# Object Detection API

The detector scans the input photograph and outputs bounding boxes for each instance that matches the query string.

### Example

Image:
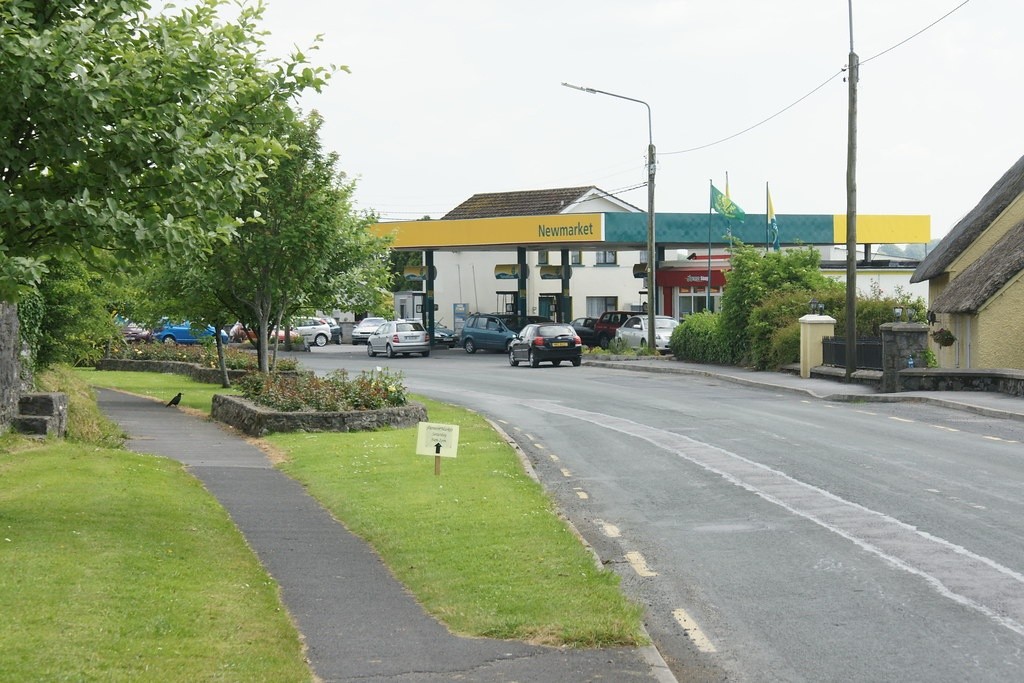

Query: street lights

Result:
[561,82,658,356]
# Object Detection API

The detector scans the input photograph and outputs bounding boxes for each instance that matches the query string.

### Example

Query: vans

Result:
[458,313,554,354]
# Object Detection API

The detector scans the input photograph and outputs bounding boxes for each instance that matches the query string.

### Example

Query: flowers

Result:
[929,327,956,342]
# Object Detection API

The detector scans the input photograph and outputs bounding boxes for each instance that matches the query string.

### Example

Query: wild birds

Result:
[166,392,184,408]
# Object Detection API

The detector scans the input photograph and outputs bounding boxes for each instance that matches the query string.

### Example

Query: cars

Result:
[153,316,229,348]
[507,321,583,368]
[116,317,150,342]
[569,317,613,348]
[351,316,388,346]
[614,314,683,355]
[366,320,431,358]
[404,317,460,348]
[228,312,344,351]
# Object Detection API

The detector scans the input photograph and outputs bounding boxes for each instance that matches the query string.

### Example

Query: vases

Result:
[932,338,954,346]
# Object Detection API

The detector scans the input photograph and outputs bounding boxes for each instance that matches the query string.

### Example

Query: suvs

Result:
[593,311,648,350]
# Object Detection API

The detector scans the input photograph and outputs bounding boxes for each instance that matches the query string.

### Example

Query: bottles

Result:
[908,354,914,368]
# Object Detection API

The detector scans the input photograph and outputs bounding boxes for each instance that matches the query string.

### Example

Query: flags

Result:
[720,184,732,238]
[712,185,745,221]
[767,189,780,252]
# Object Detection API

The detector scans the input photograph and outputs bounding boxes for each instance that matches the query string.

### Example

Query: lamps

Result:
[818,301,825,315]
[892,305,902,320]
[907,305,915,324]
[809,298,818,314]
